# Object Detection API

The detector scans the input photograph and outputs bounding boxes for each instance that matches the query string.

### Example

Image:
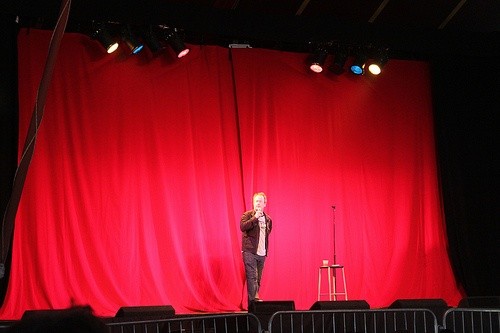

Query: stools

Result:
[318,266,348,302]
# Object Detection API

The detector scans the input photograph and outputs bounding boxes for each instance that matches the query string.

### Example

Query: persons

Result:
[240,192,272,302]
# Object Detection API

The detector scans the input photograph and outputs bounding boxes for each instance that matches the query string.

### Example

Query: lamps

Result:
[114,25,145,55]
[329,44,350,73]
[349,47,368,74]
[159,25,190,58]
[90,21,119,54]
[141,26,165,53]
[368,44,392,75]
[309,47,327,73]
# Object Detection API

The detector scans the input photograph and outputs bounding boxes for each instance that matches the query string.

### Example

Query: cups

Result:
[322,260,328,267]
